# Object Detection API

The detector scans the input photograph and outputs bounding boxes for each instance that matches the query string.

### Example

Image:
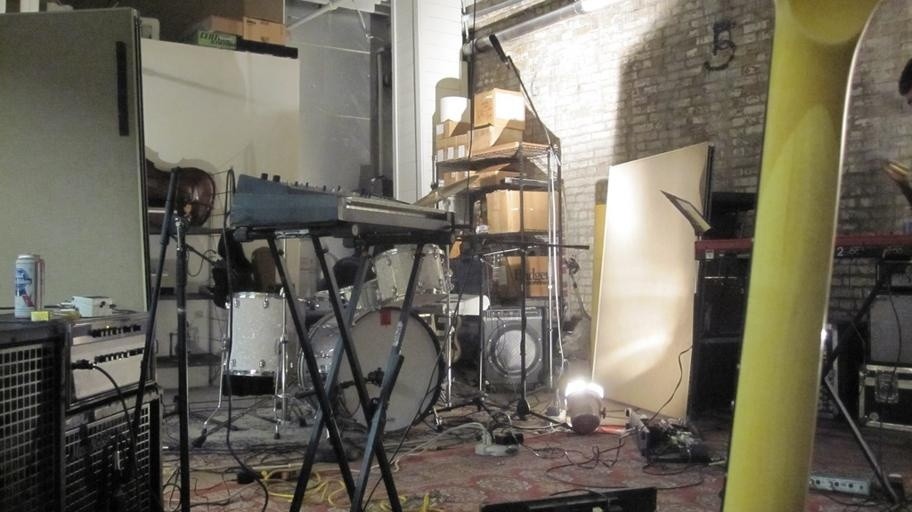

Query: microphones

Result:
[485,29,513,71]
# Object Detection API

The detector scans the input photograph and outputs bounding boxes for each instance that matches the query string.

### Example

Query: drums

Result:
[297,303,442,432]
[220,290,307,378]
[371,243,449,308]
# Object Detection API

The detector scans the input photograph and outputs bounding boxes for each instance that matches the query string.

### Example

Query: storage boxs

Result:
[241,16,288,45]
[497,256,551,297]
[436,139,445,161]
[472,126,523,150]
[486,190,551,234]
[857,363,911,431]
[474,88,526,129]
[446,136,457,160]
[183,16,244,36]
[186,28,236,50]
[469,163,520,188]
[435,119,471,139]
[456,134,468,160]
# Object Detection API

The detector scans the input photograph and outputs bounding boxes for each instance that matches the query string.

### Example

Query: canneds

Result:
[12,254,41,319]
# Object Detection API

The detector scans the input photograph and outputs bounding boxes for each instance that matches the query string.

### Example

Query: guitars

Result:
[338,279,376,308]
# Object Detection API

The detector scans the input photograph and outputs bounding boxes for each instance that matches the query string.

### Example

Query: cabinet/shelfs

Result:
[433,141,555,397]
[147,169,237,389]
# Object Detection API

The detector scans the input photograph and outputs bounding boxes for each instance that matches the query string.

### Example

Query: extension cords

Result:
[470,437,520,459]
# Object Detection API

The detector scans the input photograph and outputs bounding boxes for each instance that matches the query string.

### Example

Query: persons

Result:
[881,59,912,211]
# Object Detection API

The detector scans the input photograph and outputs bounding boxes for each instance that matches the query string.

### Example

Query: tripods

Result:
[431,260,511,433]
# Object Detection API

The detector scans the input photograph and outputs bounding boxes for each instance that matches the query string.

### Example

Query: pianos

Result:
[230,173,454,237]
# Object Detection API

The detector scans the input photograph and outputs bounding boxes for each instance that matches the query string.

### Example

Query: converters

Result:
[810,465,877,497]
